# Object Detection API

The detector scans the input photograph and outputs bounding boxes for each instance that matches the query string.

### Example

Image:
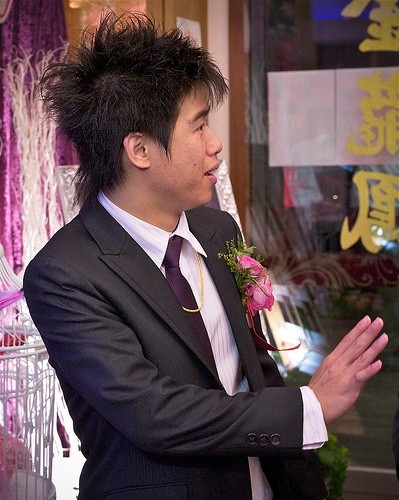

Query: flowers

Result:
[217,233,276,317]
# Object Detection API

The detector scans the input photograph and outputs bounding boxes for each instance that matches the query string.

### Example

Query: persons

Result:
[23,10,388,500]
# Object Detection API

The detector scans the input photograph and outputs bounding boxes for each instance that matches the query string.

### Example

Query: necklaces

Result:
[182,250,204,313]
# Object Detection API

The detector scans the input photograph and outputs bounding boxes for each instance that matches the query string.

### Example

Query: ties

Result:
[163,235,215,365]
